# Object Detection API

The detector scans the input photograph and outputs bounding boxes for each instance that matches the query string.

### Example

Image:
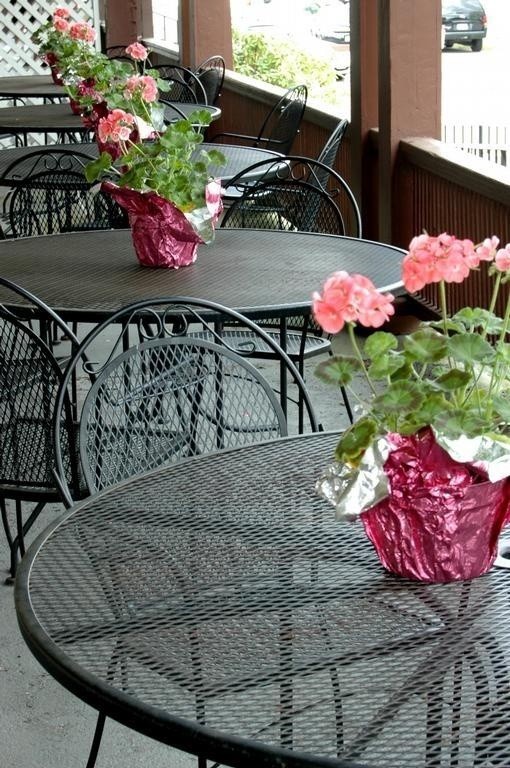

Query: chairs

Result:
[52,294,447,768]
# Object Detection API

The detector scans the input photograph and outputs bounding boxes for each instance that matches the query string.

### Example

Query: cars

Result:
[240,1,487,78]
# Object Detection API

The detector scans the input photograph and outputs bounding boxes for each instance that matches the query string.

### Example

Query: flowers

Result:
[33,8,97,57]
[311,223,510,469]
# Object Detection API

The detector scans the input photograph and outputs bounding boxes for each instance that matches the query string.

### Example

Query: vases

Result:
[322,431,510,584]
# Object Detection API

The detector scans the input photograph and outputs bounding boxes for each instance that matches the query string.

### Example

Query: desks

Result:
[0,226,419,576]
[13,427,510,767]
